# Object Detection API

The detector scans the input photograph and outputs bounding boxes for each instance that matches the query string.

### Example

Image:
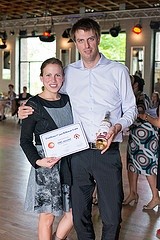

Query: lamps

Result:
[11,30,14,36]
[133,18,142,34]
[45,26,52,36]
[150,18,160,29]
[62,28,71,38]
[110,20,121,37]
[19,27,27,35]
[31,27,39,36]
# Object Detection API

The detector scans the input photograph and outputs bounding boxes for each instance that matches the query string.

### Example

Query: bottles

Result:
[96,111,112,150]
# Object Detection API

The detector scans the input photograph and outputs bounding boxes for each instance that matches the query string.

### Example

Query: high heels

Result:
[143,199,160,210]
[122,193,139,205]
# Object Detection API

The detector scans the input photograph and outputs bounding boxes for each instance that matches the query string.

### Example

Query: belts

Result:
[87,142,119,150]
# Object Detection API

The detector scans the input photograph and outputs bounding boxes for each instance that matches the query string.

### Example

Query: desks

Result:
[0,100,11,120]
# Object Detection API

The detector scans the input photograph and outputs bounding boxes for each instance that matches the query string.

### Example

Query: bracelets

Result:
[145,114,150,122]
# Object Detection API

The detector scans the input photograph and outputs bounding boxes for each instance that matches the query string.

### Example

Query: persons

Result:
[156,229,160,239]
[17,86,32,107]
[19,58,74,240]
[123,70,160,210]
[6,83,16,105]
[17,16,138,240]
